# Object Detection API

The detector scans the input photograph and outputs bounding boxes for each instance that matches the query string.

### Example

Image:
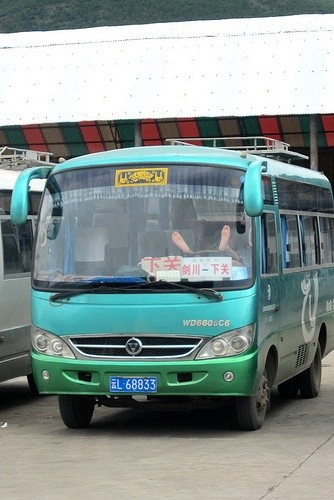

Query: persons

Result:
[171,224,248,262]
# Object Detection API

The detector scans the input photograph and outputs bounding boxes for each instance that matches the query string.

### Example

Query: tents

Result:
[0,12,334,173]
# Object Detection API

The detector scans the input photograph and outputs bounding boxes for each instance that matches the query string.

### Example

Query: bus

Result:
[8,134,334,433]
[0,146,65,400]
